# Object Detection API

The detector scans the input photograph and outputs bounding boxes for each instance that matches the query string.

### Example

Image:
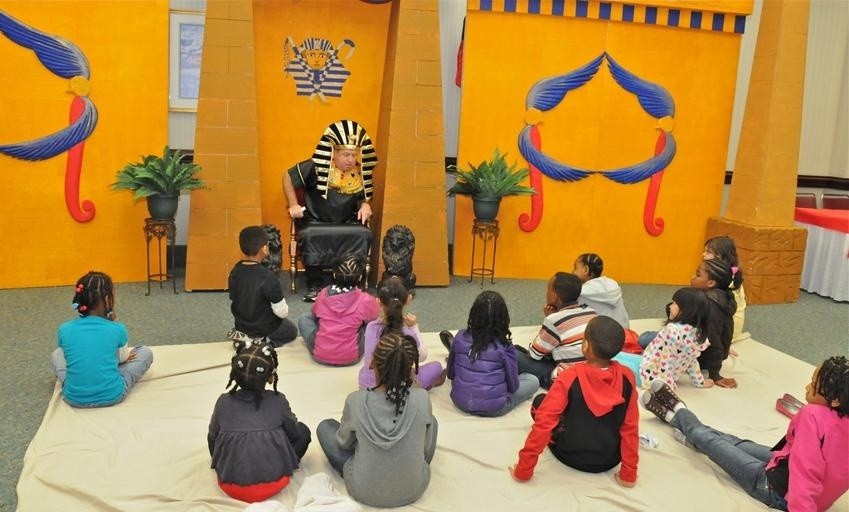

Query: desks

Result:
[793,206,848,303]
[143,217,179,297]
[467,218,500,290]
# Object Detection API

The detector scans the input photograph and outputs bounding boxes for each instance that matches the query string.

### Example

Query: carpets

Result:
[14,314,849,512]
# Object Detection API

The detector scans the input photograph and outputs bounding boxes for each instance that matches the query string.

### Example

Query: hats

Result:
[312,120,377,203]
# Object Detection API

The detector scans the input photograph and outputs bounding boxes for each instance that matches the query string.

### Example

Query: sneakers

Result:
[302,286,322,302]
[227,328,271,350]
[639,379,686,424]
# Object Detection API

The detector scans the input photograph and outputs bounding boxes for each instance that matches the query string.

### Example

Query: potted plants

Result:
[107,144,208,222]
[445,143,538,222]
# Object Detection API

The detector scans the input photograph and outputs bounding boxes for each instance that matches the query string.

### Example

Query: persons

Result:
[511,271,596,390]
[227,226,298,352]
[283,119,378,303]
[609,287,714,395]
[297,256,447,508]
[638,260,737,388]
[52,272,153,410]
[207,338,313,503]
[638,356,849,511]
[509,316,639,488]
[447,291,539,420]
[573,253,630,343]
[702,236,746,357]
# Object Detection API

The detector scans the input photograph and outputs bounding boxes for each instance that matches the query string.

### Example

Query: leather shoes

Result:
[777,393,805,419]
[440,330,455,352]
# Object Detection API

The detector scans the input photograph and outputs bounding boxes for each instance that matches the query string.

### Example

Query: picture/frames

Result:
[167,7,206,114]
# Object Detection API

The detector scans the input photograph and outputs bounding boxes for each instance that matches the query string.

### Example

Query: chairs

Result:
[819,192,848,210]
[289,185,373,296]
[795,191,817,209]
